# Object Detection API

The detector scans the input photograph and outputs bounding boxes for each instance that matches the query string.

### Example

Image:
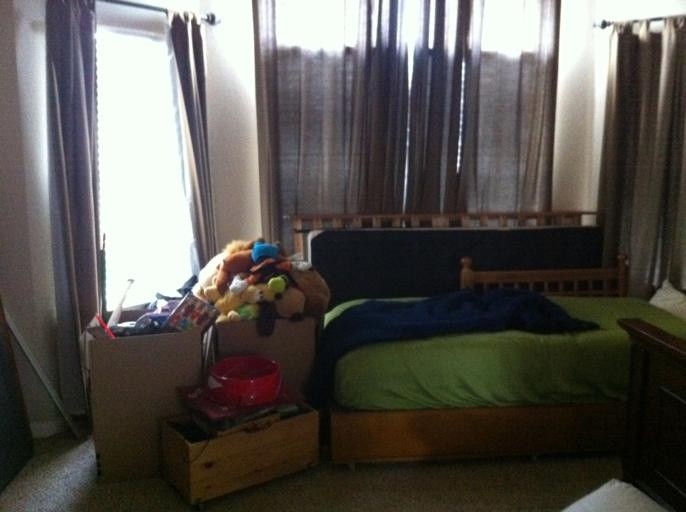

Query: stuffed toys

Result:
[192,239,332,324]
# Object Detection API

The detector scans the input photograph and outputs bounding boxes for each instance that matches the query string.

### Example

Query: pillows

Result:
[648,277,686,321]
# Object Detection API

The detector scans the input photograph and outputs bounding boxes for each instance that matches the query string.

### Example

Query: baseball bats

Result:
[107,278,134,329]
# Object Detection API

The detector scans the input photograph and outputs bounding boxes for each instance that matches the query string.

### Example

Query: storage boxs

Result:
[82,320,204,484]
[161,398,320,506]
[203,315,317,399]
[102,307,156,325]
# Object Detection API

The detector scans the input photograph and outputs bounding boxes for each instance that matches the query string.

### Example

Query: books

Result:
[163,292,221,337]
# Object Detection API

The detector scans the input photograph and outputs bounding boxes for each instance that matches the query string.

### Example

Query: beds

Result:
[323,293,686,464]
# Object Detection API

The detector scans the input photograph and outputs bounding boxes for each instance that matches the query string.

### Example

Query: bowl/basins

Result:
[209,355,283,403]
